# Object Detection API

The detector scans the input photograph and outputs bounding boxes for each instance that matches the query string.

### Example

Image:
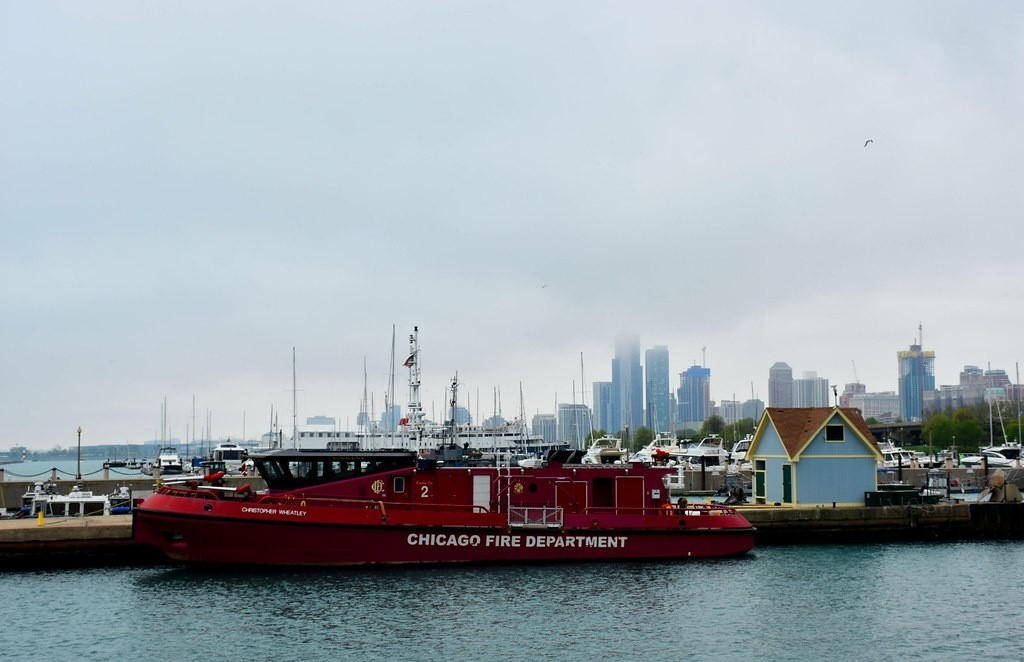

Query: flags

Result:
[404,354,415,368]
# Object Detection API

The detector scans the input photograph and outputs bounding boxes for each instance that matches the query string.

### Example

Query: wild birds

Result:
[864,138,875,147]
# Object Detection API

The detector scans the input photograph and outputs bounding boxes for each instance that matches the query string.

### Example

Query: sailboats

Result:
[1,322,1023,567]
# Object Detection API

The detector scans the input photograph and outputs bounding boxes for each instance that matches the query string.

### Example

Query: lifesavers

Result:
[658,504,676,515]
[280,494,298,505]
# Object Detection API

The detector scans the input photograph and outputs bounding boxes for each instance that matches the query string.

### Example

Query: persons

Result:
[739,487,743,500]
[932,479,937,487]
[208,468,228,498]
[20,500,34,516]
[716,485,727,496]
[967,480,971,486]
